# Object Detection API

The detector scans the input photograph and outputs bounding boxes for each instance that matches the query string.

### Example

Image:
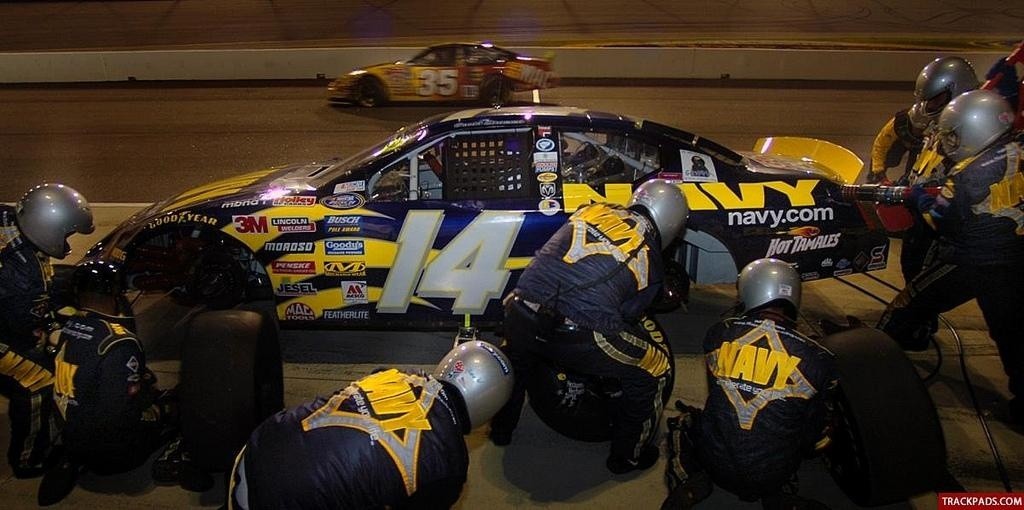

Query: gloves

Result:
[908,184,936,211]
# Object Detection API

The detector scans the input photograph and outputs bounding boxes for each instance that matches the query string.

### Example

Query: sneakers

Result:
[764,489,829,509]
[660,474,715,509]
[607,443,660,474]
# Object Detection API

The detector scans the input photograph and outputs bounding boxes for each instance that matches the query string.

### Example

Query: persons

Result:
[427,48,452,65]
[861,49,986,355]
[31,261,206,510]
[659,256,858,508]
[870,53,1023,437]
[0,182,95,482]
[484,176,689,477]
[223,341,512,509]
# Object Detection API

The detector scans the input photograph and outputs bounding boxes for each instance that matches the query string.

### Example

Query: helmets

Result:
[68,260,127,296]
[736,256,802,322]
[913,55,981,123]
[625,179,690,252]
[434,341,515,436]
[937,89,1014,163]
[17,184,94,261]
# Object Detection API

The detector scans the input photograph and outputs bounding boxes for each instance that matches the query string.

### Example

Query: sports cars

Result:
[73,103,893,335]
[325,40,556,109]
